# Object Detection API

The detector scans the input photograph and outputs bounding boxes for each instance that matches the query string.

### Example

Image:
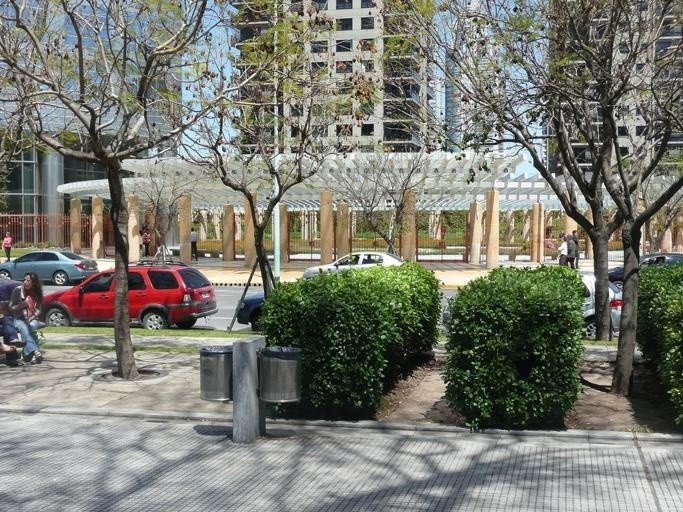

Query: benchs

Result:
[0,301,47,339]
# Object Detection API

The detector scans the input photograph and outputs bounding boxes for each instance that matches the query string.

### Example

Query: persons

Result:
[1,231,13,262]
[142,229,152,257]
[190,227,197,261]
[545,228,621,270]
[138,230,143,250]
[0,271,46,367]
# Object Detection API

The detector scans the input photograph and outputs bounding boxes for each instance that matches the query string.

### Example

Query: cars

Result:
[0,277,23,315]
[0,250,99,286]
[234,294,265,333]
[606,253,682,291]
[300,251,405,279]
[576,271,623,342]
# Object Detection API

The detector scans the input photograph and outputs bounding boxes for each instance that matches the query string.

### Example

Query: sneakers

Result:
[30,352,43,364]
[0,345,16,354]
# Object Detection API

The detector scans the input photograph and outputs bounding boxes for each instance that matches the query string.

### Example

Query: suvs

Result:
[36,260,218,331]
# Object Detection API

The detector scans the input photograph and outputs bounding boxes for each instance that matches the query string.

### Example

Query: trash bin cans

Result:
[200,336,302,443]
